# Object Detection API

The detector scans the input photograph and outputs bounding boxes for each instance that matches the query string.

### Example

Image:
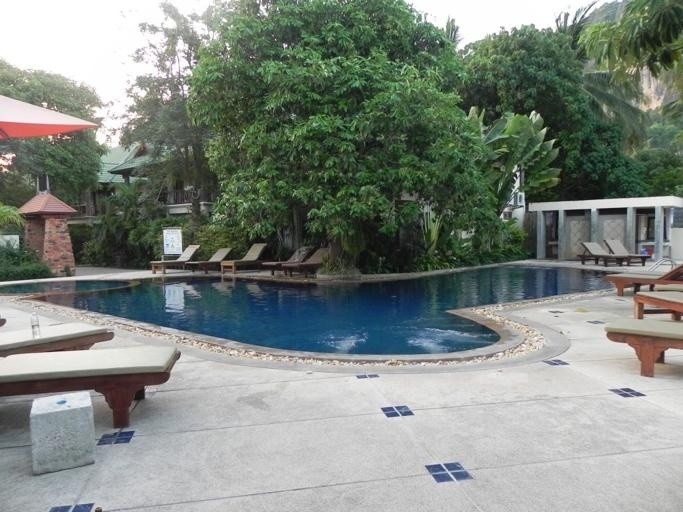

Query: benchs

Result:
[602,282,682,377]
[0,320,182,428]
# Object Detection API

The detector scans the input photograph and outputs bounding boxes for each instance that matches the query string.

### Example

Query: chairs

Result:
[575,239,651,267]
[601,263,682,297]
[150,242,330,276]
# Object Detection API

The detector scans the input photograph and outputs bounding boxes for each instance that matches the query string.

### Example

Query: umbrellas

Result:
[0,94,99,140]
